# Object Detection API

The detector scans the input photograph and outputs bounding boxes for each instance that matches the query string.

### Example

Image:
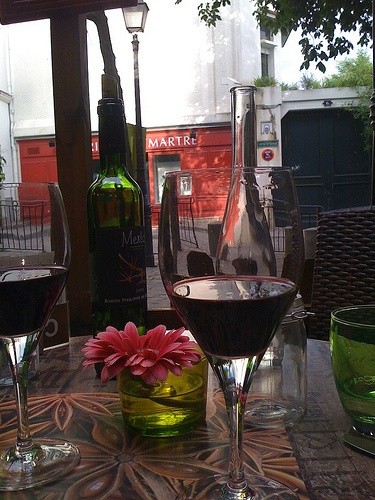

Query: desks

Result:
[0,336,375,500]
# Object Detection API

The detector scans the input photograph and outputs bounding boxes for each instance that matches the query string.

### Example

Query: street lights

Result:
[122,1,156,266]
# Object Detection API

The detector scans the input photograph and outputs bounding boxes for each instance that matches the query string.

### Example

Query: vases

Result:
[119,343,208,438]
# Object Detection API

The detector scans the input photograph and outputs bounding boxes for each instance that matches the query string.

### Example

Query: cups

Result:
[328,302,374,440]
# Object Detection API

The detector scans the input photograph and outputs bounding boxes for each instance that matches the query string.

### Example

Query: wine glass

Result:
[0,181,82,494]
[158,169,303,500]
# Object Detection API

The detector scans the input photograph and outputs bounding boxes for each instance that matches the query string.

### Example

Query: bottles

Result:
[210,82,288,430]
[88,98,149,379]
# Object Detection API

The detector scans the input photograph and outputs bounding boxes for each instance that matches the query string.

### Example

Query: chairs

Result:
[306,207,375,343]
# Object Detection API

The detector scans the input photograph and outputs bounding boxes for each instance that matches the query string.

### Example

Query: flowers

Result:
[81,323,199,384]
